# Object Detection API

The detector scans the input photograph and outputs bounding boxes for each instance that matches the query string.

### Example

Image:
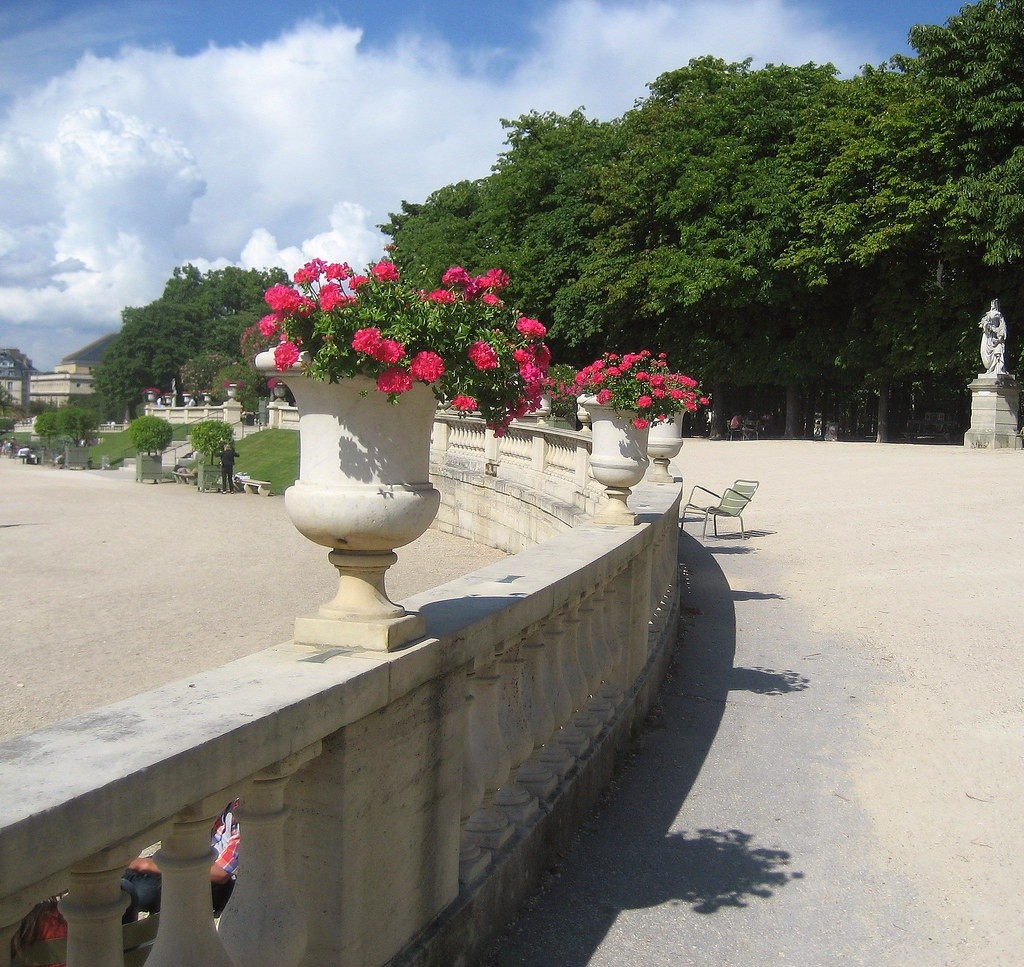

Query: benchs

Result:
[232,475,271,498]
[727,419,760,440]
[172,470,197,484]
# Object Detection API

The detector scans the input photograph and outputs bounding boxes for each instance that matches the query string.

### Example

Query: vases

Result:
[273,383,287,402]
[648,398,689,485]
[587,396,650,525]
[147,391,192,407]
[202,393,212,406]
[227,384,239,402]
[254,345,441,653]
[577,394,593,433]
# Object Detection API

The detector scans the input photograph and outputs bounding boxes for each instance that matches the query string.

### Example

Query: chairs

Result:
[679,479,759,540]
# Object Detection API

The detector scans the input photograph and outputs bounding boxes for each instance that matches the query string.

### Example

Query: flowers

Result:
[259,258,551,439]
[202,389,212,393]
[144,387,161,395]
[223,378,245,390]
[266,377,282,390]
[534,392,553,429]
[544,364,710,429]
[164,392,173,396]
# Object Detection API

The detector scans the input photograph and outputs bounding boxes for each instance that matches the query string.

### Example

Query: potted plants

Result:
[191,420,234,492]
[129,415,173,483]
[35,408,99,470]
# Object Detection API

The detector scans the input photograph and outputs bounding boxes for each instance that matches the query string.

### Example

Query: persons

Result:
[174,464,198,474]
[980,299,1008,374]
[14,798,243,944]
[218,443,240,494]
[0,436,32,463]
[731,412,752,440]
